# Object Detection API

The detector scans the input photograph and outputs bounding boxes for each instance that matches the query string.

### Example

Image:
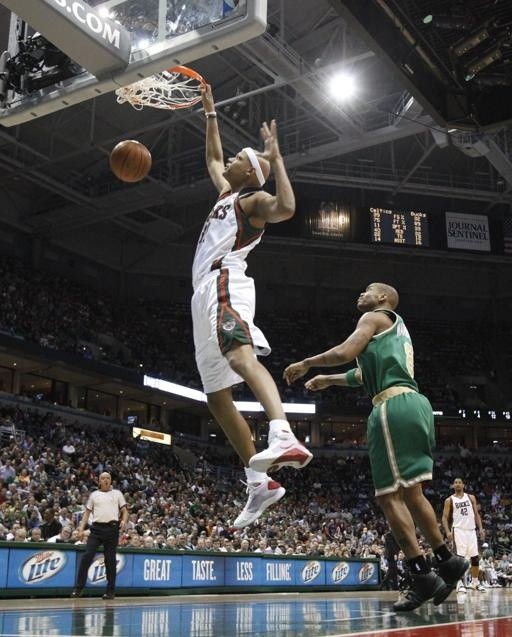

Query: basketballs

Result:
[110,139,151,182]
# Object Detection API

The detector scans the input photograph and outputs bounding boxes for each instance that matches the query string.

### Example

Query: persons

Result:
[441,475,486,594]
[1,219,511,585]
[282,280,469,616]
[189,73,315,532]
[68,466,130,599]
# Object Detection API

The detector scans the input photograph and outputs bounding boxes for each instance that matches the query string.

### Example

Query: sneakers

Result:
[393,554,469,611]
[233,478,286,528]
[248,433,313,472]
[72,591,82,597]
[102,592,115,599]
[475,583,487,593]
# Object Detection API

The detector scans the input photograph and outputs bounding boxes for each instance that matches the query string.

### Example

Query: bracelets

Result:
[203,111,218,118]
